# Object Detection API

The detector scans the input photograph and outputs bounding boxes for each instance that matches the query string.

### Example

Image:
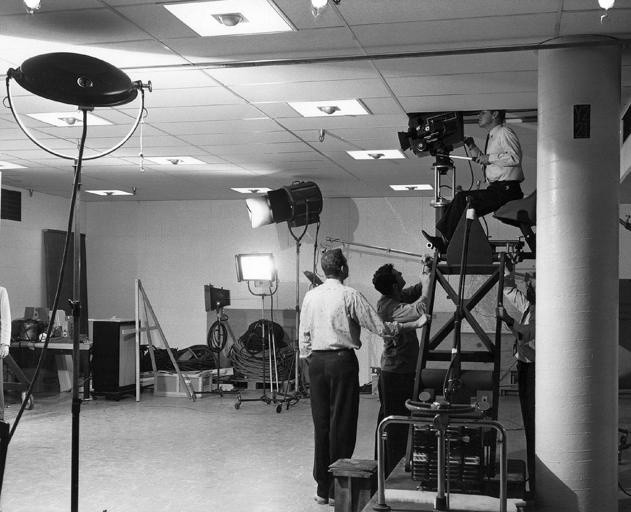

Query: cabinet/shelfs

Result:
[87,318,143,405]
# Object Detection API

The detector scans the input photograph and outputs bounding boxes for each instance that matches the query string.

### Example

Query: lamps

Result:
[233,251,288,412]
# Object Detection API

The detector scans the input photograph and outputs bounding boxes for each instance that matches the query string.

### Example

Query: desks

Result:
[4,334,94,399]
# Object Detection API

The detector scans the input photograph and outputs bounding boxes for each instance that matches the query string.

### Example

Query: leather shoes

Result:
[422,230,446,255]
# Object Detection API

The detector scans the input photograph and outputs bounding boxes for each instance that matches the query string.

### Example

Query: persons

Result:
[297,247,431,505]
[0,285,14,422]
[372,261,431,489]
[420,109,524,254]
[497,257,536,498]
